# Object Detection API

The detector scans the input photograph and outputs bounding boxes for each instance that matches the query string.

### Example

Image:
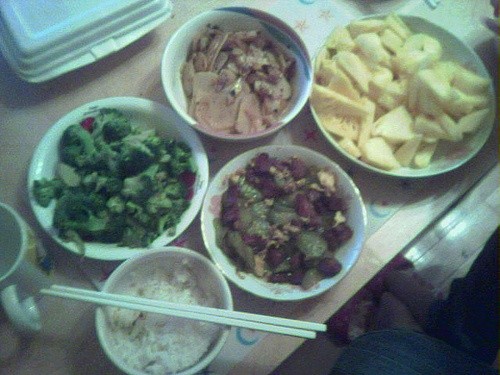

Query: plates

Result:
[28,96,208,260]
[200,143,367,301]
[309,13,497,178]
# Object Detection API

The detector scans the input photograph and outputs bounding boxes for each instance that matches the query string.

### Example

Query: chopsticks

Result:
[40,284,327,339]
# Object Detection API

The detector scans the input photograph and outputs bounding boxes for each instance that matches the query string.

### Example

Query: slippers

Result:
[367,254,443,309]
[324,288,383,344]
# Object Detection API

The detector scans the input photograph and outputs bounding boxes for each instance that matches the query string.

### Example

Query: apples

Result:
[312,10,491,171]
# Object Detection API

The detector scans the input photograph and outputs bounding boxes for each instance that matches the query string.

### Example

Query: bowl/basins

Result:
[163,7,313,141]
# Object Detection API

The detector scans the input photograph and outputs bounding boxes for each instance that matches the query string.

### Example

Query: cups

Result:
[0,204,54,337]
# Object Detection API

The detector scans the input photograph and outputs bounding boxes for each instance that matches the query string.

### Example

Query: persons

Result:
[329,224,499,374]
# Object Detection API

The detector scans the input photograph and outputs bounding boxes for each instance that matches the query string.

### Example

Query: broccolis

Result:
[26,97,208,261]
